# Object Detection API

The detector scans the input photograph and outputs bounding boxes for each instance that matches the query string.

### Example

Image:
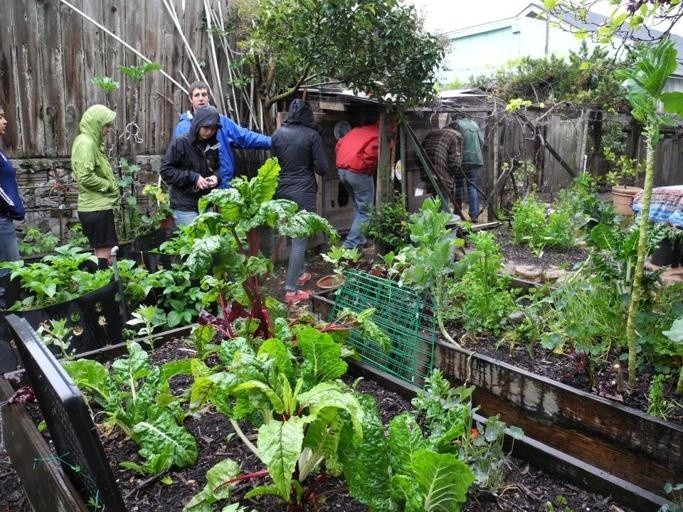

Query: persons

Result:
[452,112,484,224]
[415,122,462,213]
[0,107,26,314]
[70,103,119,265]
[271,97,328,304]
[156,105,222,233]
[334,111,396,251]
[174,80,271,189]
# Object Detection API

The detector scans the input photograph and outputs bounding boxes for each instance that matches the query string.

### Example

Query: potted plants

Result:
[0,229,119,350]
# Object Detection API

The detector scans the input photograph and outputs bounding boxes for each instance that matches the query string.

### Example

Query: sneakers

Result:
[297,272,311,286]
[285,289,314,303]
